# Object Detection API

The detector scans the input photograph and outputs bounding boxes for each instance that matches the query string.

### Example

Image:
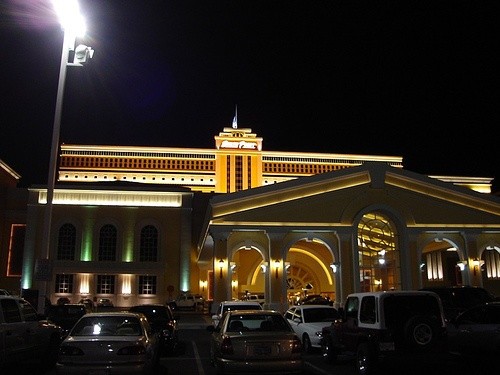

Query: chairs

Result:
[261,321,272,327]
[231,321,243,329]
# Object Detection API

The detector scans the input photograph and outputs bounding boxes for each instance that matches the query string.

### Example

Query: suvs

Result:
[1,289,63,367]
[416,285,496,324]
[319,291,446,375]
[167,293,204,311]
[127,305,178,350]
[45,303,87,329]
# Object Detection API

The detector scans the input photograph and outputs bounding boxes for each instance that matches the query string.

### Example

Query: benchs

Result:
[79,326,134,334]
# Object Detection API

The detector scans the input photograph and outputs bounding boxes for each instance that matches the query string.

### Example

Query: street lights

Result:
[37,0,95,313]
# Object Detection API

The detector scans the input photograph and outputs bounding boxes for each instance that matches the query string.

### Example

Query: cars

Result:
[283,304,342,353]
[210,309,302,375]
[211,301,272,331]
[447,296,500,364]
[96,298,113,309]
[63,312,156,375]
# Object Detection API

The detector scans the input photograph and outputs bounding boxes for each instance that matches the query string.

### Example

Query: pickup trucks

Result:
[238,294,264,305]
